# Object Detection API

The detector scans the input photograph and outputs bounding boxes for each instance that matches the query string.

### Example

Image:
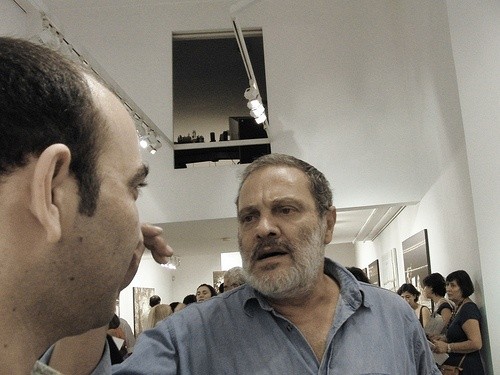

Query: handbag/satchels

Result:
[441,364,463,375]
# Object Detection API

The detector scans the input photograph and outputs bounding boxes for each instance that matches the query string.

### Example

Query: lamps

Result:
[243,79,259,101]
[249,104,266,119]
[255,113,267,125]
[246,99,262,110]
[160,255,182,270]
[24,13,163,156]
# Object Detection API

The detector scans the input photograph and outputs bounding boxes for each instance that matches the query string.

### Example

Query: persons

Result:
[0,36,149,375]
[40,154,443,375]
[396,283,431,328]
[430,270,485,375]
[423,273,455,334]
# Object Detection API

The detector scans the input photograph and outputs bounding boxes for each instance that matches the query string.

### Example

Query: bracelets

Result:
[452,343,456,353]
[447,343,451,353]
